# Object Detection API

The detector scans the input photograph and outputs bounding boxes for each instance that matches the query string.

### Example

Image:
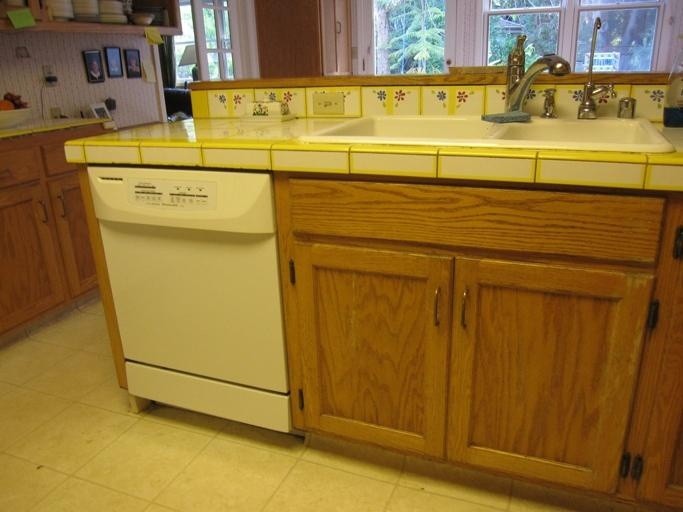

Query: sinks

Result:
[297,114,496,153]
[490,113,675,154]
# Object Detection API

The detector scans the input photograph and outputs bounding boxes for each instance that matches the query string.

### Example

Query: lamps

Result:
[178,45,199,81]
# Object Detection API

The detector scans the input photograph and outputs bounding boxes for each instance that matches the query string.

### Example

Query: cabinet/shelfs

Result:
[0,0,182,35]
[254,0,353,79]
[627,192,683,512]
[0,124,116,348]
[274,172,674,512]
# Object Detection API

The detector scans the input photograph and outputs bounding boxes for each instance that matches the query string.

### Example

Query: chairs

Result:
[165,88,192,120]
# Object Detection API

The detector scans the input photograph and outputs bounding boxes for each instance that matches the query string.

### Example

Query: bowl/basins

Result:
[0,108,33,130]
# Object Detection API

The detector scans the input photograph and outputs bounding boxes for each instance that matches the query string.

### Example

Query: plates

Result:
[2,0,165,25]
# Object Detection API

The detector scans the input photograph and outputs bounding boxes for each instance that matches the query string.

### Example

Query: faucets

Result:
[506,32,573,113]
[577,15,617,120]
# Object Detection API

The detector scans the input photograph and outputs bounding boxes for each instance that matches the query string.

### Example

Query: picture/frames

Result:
[82,47,142,83]
[90,102,115,129]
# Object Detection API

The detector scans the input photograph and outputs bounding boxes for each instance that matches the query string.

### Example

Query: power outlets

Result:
[43,65,57,86]
